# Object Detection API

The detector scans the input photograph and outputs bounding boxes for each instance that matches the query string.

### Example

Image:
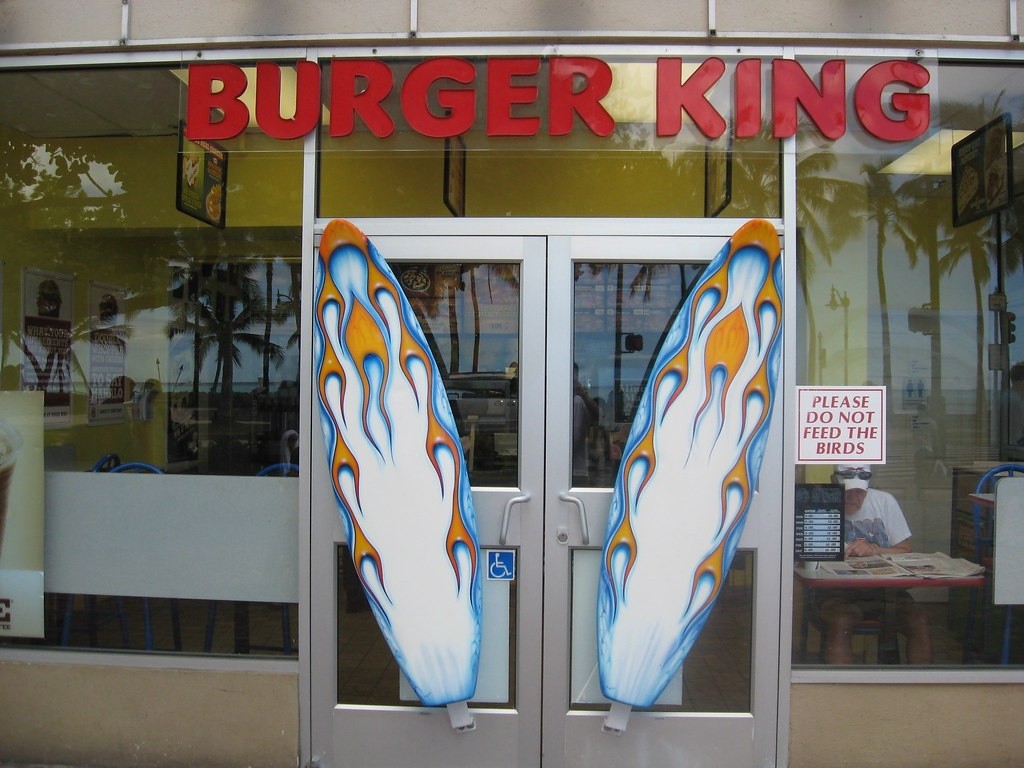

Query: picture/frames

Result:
[172,262,252,324]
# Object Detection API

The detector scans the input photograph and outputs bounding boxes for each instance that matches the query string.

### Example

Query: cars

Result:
[442,370,589,466]
[607,380,646,446]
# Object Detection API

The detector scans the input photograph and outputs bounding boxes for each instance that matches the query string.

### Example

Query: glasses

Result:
[834,470,872,480]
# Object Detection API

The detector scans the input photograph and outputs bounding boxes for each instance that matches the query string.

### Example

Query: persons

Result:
[572,362,591,487]
[810,462,932,665]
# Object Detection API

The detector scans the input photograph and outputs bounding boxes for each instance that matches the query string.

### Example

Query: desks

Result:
[461,416,517,474]
[794,556,986,664]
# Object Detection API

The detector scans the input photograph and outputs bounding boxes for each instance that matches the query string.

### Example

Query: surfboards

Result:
[310,219,488,735]
[593,218,786,739]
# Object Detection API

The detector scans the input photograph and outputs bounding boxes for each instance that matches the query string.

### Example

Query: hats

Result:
[833,463,871,492]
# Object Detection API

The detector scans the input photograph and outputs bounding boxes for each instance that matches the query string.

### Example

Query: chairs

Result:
[962,463,1024,664]
[799,583,888,665]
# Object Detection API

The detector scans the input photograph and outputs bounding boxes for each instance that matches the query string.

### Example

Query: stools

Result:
[58,453,300,654]
[947,465,1023,664]
